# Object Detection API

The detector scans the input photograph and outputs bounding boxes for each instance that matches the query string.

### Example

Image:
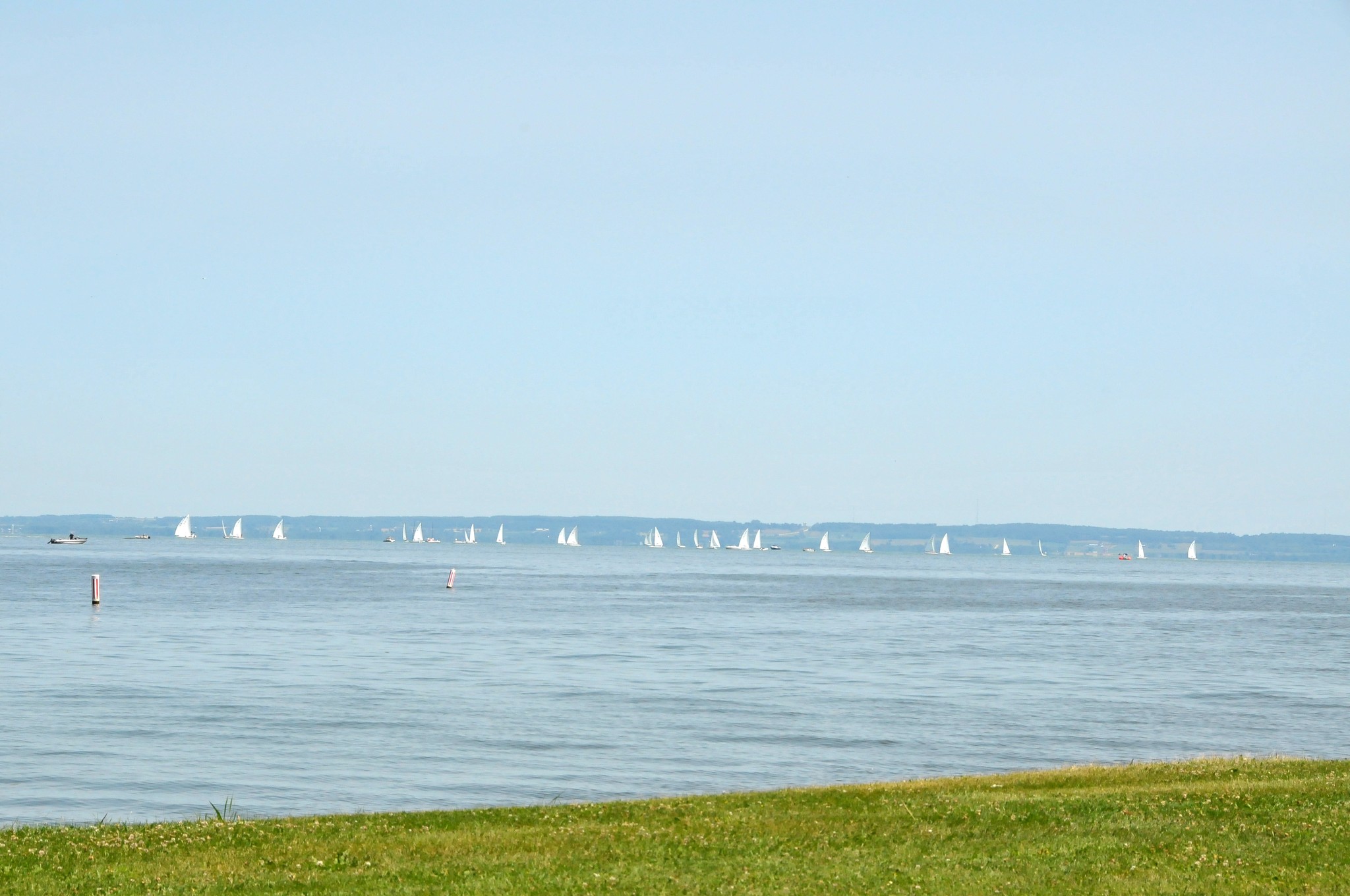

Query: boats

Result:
[135,533,148,539]
[47,536,88,544]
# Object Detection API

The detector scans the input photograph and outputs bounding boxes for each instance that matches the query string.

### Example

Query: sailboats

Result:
[175,515,1202,562]
